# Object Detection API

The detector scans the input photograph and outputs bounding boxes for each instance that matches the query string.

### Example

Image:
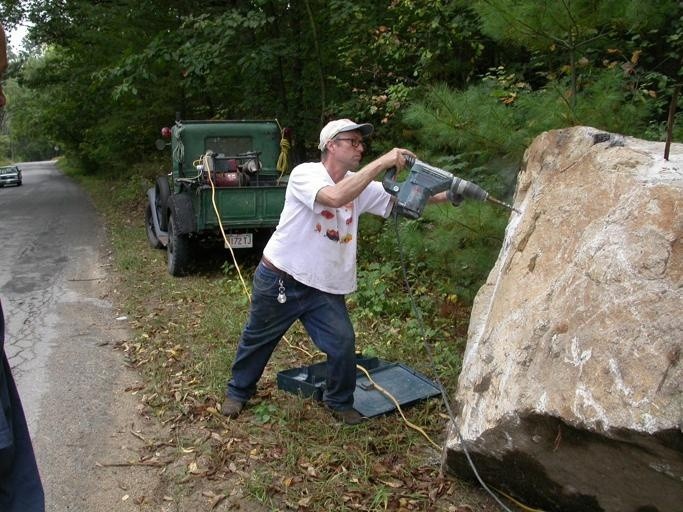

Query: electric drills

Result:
[383,153,523,220]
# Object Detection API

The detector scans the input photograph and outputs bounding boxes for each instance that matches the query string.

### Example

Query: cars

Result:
[0,165,23,188]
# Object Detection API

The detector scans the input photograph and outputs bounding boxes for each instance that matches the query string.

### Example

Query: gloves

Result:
[446,179,474,207]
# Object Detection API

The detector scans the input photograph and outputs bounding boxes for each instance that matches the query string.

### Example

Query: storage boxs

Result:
[277,355,442,420]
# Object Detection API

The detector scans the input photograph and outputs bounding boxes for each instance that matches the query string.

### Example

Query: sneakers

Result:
[221,395,247,421]
[323,401,363,425]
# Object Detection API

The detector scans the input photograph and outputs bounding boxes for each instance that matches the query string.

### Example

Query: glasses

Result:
[334,138,365,148]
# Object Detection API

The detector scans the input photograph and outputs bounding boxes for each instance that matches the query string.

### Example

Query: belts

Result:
[261,252,286,273]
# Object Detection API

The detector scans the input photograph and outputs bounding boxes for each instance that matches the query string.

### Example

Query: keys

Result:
[277,280,286,304]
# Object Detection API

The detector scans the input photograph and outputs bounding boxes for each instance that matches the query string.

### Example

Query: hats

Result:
[317,118,375,152]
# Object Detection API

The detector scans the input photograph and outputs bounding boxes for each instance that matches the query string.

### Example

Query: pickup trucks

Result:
[142,117,295,284]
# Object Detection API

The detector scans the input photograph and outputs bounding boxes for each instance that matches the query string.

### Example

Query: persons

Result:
[0,28,46,512]
[221,119,448,424]
